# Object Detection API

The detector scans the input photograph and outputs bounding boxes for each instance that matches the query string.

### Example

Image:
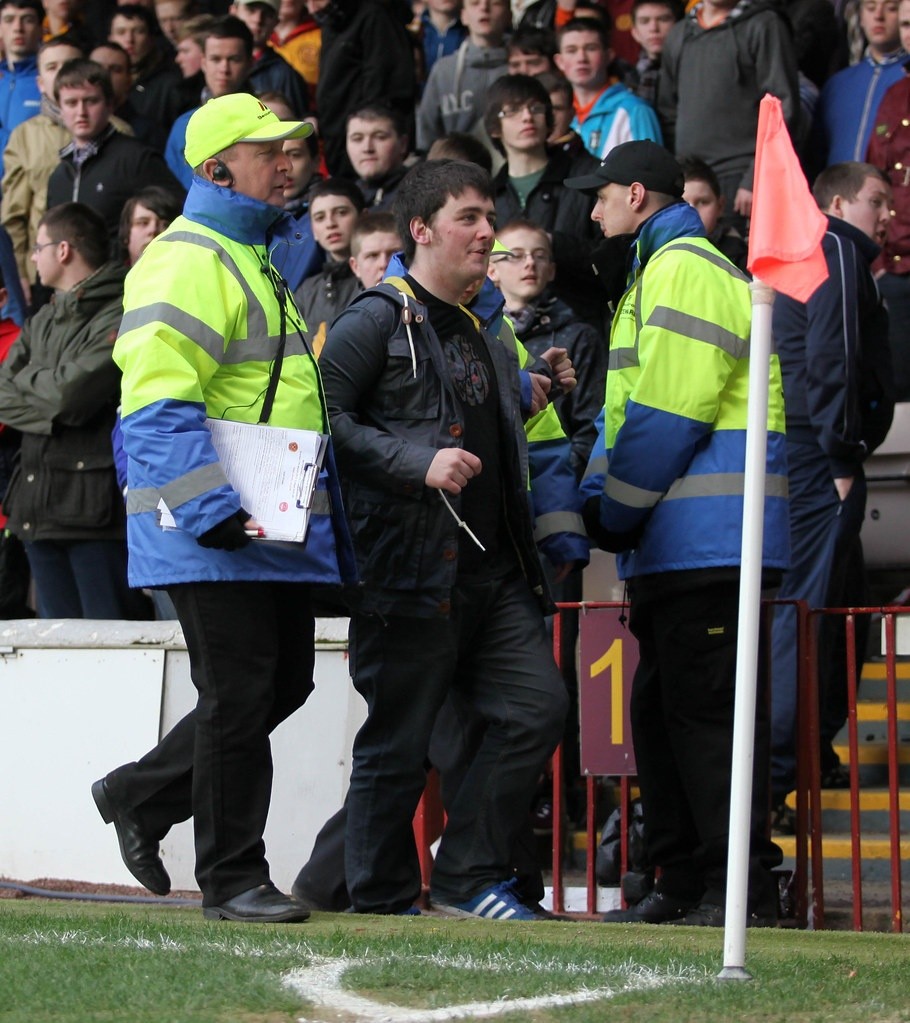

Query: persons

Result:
[578,137,795,926]
[0,0,910,911]
[93,88,358,919]
[289,155,578,921]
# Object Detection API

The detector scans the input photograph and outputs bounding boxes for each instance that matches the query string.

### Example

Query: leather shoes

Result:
[91,777,171,896]
[204,884,311,922]
[603,889,778,928]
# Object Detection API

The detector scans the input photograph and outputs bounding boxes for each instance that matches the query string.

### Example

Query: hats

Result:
[563,138,686,197]
[184,93,314,169]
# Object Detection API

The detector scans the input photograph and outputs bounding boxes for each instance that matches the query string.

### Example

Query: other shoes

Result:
[820,765,872,789]
[526,903,576,922]
[291,885,327,911]
[772,804,795,834]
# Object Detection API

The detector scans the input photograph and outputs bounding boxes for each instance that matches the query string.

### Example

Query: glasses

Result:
[33,241,79,254]
[492,250,551,264]
[496,104,547,118]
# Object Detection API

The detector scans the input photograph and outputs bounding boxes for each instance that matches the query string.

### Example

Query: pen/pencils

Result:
[244,529,264,537]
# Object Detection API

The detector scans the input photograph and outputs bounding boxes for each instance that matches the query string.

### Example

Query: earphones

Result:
[213,161,227,181]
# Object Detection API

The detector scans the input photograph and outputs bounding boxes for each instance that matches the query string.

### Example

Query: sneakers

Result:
[430,877,545,921]
